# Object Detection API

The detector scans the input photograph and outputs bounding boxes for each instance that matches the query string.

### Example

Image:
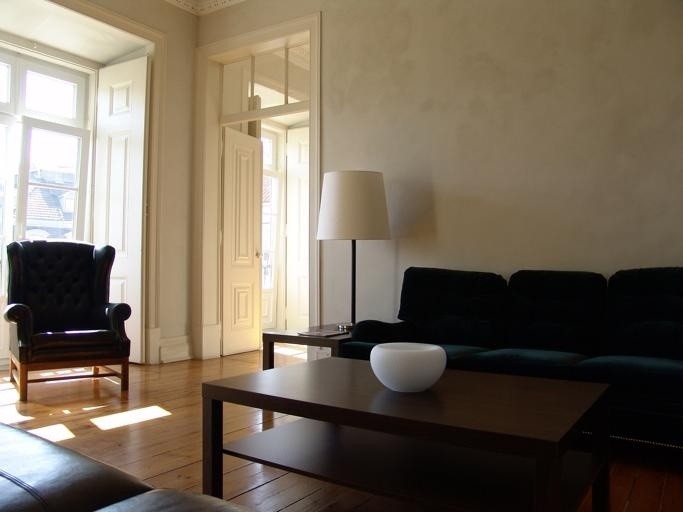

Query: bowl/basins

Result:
[369,342,448,394]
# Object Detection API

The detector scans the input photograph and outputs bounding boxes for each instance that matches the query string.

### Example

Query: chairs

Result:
[3,239,132,403]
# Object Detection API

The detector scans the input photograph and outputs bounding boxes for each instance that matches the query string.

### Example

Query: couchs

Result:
[340,263,682,470]
[0,422,241,511]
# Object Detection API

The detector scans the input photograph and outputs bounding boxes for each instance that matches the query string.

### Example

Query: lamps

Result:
[311,168,394,328]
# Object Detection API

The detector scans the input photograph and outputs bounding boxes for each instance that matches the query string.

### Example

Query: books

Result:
[299,326,349,339]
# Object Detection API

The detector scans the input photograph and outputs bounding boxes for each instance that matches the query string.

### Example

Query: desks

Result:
[257,324,354,370]
